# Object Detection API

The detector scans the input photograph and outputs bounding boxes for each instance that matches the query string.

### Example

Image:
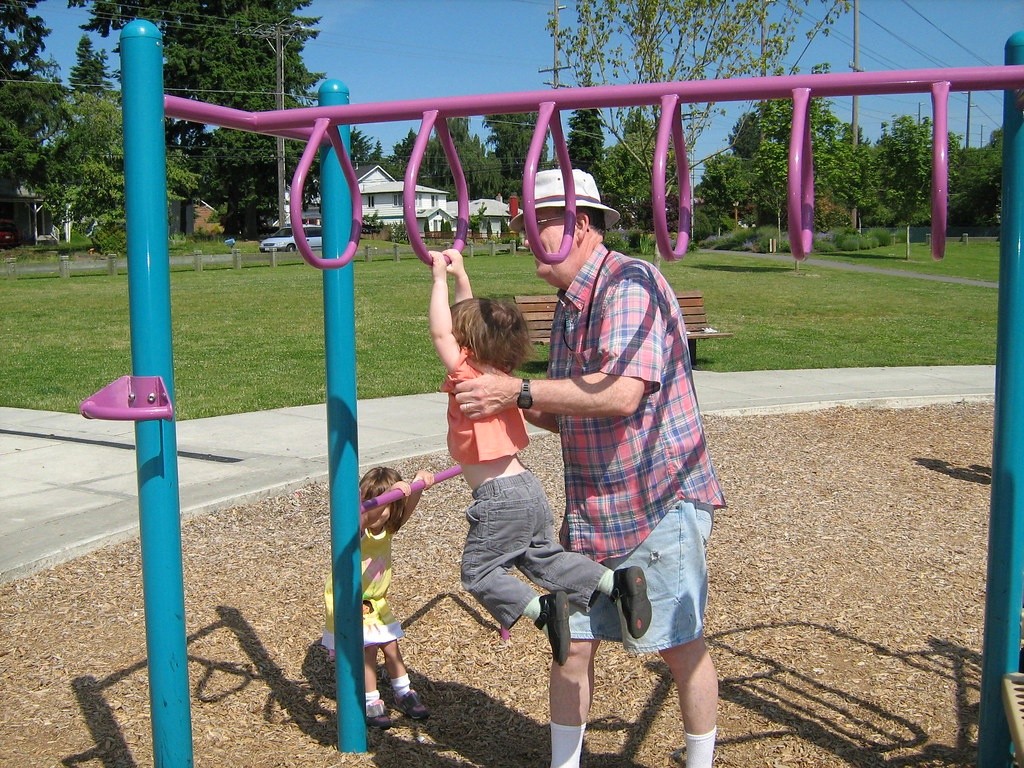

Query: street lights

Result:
[732,200,740,233]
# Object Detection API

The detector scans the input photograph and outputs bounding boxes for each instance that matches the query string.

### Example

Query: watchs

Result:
[516,379,533,409]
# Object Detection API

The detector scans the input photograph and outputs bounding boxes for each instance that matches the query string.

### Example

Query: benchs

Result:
[514,290,734,370]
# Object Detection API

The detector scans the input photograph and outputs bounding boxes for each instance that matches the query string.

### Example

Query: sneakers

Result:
[393,689,430,719]
[366,699,392,727]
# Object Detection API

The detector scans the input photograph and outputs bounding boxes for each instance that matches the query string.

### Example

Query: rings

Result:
[464,404,467,412]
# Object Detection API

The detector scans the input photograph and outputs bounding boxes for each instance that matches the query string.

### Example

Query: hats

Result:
[509,169,620,233]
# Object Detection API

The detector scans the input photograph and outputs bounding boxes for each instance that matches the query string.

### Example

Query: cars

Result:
[0,217,22,250]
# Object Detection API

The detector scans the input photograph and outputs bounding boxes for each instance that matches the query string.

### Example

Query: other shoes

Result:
[609,566,652,639]
[534,590,571,666]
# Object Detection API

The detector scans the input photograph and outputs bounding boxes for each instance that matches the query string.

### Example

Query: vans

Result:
[258,222,322,254]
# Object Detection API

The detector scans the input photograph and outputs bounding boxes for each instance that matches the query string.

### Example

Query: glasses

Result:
[524,216,564,232]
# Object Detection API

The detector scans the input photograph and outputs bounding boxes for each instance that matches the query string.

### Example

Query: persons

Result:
[321,467,434,729]
[456,167,730,768]
[429,248,653,666]
